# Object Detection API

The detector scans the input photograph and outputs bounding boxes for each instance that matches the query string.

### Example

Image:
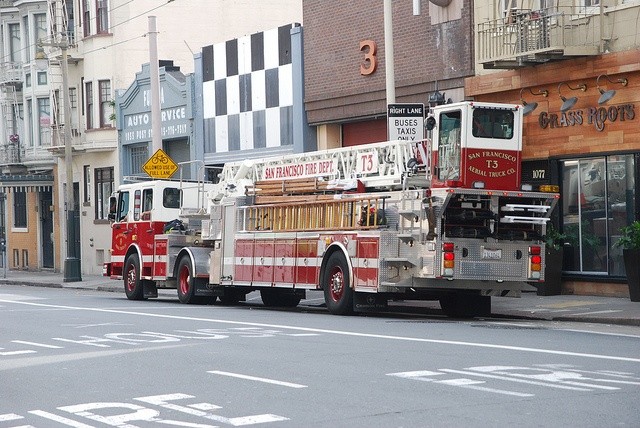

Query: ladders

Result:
[236,195,391,233]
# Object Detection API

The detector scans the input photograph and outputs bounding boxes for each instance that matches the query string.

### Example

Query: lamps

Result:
[519,86,549,115]
[595,74,628,105]
[557,80,587,111]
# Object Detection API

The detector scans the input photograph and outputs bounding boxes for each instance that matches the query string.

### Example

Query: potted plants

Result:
[611,221,640,302]
[565,218,601,270]
[537,226,566,295]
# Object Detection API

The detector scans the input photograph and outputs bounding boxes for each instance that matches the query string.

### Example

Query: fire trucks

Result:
[102,100,562,316]
